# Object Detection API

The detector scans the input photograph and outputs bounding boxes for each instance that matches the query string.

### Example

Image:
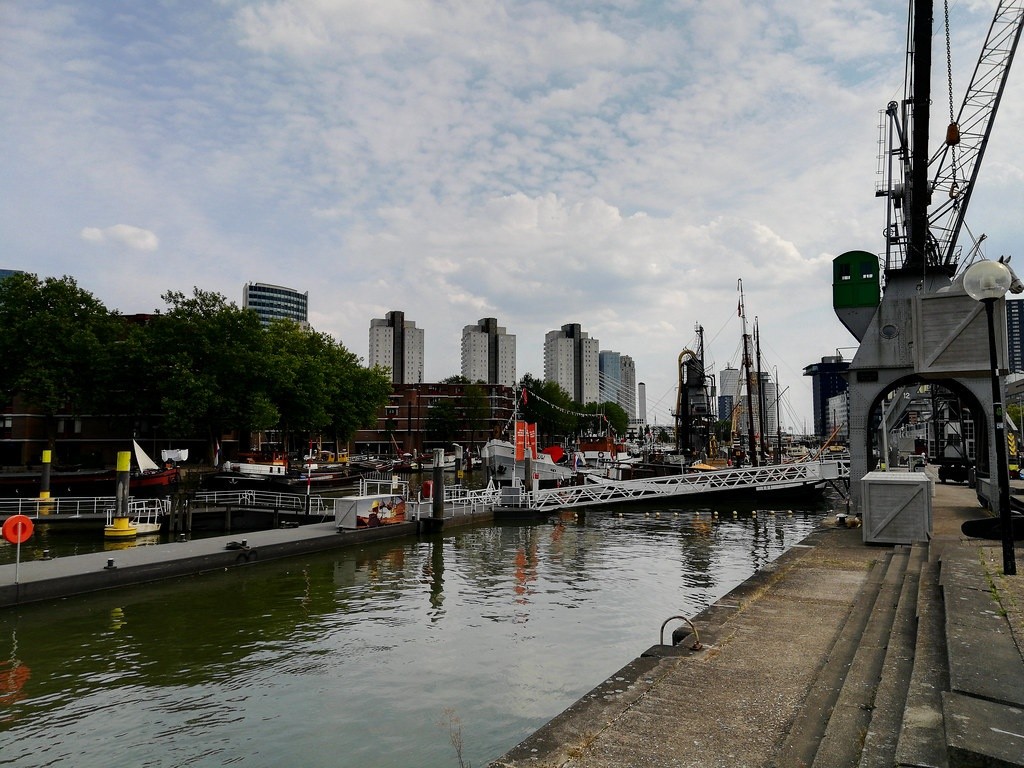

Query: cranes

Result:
[825,0,1024,524]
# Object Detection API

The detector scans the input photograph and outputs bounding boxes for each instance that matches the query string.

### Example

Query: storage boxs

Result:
[860,471,933,543]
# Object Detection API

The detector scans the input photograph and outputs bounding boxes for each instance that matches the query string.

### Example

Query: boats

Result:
[479,437,573,486]
[302,435,484,472]
[105,505,162,536]
[570,274,847,489]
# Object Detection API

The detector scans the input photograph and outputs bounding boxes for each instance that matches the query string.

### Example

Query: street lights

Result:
[960,260,1022,576]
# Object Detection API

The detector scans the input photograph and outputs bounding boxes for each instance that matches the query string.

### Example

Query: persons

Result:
[368,501,380,527]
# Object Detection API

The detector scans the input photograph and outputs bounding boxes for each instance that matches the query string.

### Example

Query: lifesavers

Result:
[598,452,604,458]
[582,452,585,457]
